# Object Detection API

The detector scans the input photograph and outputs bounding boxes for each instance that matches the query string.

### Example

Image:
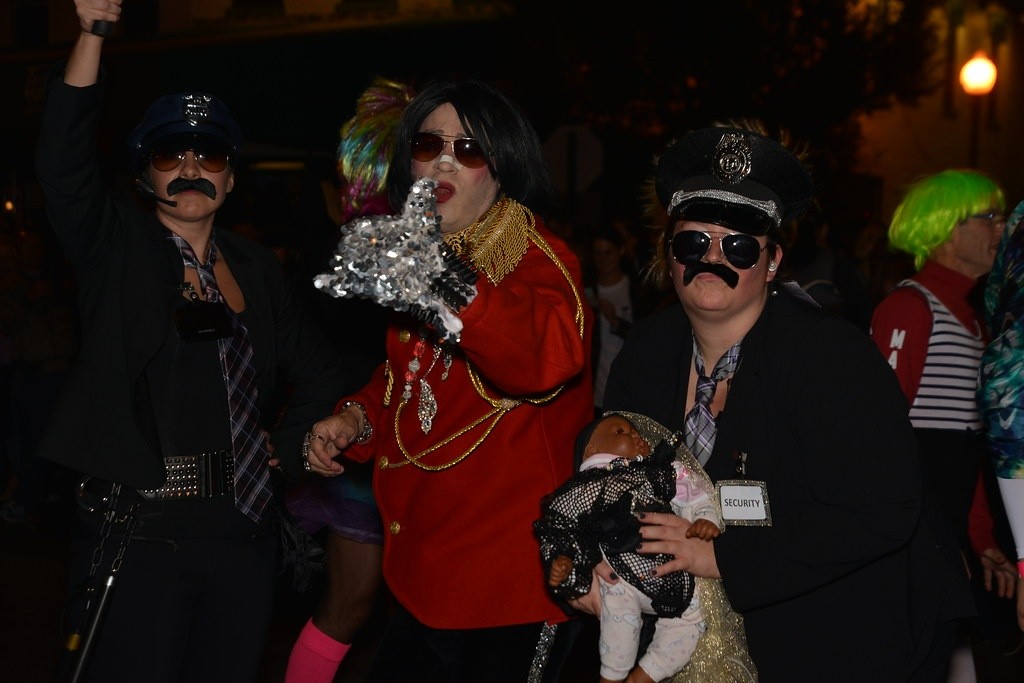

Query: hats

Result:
[126,91,243,165]
[655,129,819,252]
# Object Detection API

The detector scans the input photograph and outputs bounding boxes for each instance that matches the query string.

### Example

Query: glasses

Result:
[972,210,1002,226]
[664,229,768,269]
[144,135,230,173]
[409,131,491,169]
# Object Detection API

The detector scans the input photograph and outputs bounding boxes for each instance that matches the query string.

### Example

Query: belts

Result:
[134,446,234,504]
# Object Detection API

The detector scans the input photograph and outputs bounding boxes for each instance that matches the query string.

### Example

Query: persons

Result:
[0,228,71,525]
[600,127,925,683]
[535,413,724,683]
[584,225,647,417]
[870,170,1019,683]
[34,1,349,683]
[283,79,416,682]
[301,81,597,682]
[980,201,1024,636]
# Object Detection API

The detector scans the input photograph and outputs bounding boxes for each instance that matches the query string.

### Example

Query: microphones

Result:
[135,175,177,207]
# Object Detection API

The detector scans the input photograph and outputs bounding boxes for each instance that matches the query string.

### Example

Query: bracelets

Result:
[1017,561,1024,578]
[342,402,373,443]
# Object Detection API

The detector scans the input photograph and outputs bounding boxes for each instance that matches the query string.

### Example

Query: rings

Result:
[304,461,312,472]
[305,433,325,441]
[302,441,311,456]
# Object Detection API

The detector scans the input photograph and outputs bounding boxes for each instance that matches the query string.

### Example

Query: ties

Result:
[177,228,279,525]
[687,334,741,467]
